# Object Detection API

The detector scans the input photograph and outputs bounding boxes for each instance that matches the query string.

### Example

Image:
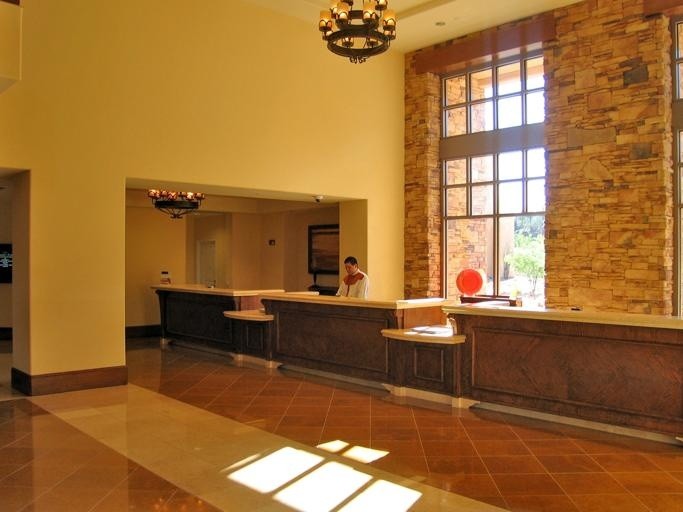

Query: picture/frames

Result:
[307,224,338,276]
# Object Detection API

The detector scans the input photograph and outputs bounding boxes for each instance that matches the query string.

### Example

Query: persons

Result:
[336,256,368,299]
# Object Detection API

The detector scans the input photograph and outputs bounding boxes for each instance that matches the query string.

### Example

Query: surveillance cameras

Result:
[315,196,324,203]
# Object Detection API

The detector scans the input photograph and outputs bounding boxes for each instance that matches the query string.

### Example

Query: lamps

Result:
[147,188,204,223]
[318,0,396,65]
